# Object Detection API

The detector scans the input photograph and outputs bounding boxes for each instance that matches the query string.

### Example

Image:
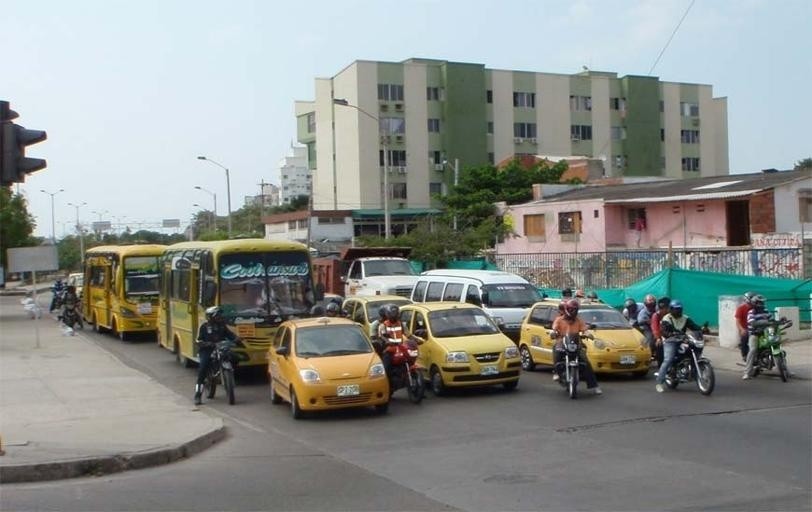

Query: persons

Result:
[370,305,386,354]
[575,290,584,298]
[623,299,645,326]
[327,303,341,317]
[656,300,711,393]
[562,288,572,298]
[194,306,241,405]
[552,301,587,374]
[550,300,603,395]
[587,291,597,299]
[50,276,85,329]
[651,297,671,370]
[379,303,424,375]
[742,294,796,380]
[735,291,756,363]
[330,297,350,318]
[633,217,645,248]
[310,304,326,318]
[637,295,660,360]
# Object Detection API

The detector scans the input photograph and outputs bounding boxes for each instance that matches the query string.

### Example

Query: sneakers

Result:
[656,384,663,393]
[194,395,201,405]
[789,371,795,376]
[552,374,559,381]
[742,373,748,379]
[594,387,602,394]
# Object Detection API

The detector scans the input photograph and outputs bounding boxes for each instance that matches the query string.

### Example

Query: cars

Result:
[518,299,651,376]
[398,302,523,396]
[268,317,390,418]
[343,296,416,352]
[68,274,84,302]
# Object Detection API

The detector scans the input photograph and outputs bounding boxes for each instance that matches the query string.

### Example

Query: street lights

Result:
[112,215,127,240]
[197,156,233,240]
[194,203,211,234]
[91,210,109,242]
[40,189,64,245]
[68,202,87,230]
[445,158,462,233]
[196,186,218,236]
[192,212,200,222]
[334,98,393,246]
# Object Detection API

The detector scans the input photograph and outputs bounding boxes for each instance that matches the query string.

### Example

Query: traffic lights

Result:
[1,119,47,185]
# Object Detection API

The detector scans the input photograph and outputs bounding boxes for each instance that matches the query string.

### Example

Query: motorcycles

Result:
[552,334,595,398]
[202,341,247,406]
[53,289,65,309]
[63,302,79,330]
[381,339,428,403]
[743,320,794,381]
[654,323,715,396]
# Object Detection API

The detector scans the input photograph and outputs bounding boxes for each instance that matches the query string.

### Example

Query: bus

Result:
[82,246,169,341]
[158,238,316,373]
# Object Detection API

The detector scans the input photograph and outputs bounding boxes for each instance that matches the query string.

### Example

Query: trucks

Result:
[314,246,423,298]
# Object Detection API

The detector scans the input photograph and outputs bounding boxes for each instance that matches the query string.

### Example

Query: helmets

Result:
[751,295,767,309]
[311,305,325,317]
[379,306,387,317]
[205,307,222,321]
[644,295,657,306]
[331,298,343,307]
[744,292,756,304]
[326,303,339,315]
[562,288,572,297]
[559,302,567,315]
[670,300,683,313]
[385,305,399,321]
[625,299,635,309]
[565,301,580,318]
[588,292,597,300]
[576,290,585,298]
[658,297,671,309]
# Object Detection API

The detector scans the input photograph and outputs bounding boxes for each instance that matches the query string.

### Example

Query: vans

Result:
[411,270,544,344]
